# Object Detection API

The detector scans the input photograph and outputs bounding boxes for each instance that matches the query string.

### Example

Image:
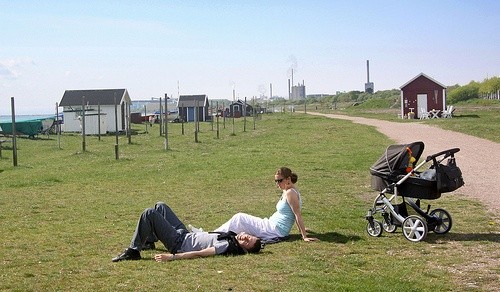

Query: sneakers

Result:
[141,241,156,251]
[111,247,141,263]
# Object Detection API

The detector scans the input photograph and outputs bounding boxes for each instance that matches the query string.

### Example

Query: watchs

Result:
[171,254,176,260]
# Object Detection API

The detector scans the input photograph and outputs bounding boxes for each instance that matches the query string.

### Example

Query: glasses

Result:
[275,178,286,183]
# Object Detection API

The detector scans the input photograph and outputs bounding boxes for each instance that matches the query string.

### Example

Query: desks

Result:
[430,110,440,119]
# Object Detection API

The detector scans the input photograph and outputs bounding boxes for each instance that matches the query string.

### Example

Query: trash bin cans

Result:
[408,112,414,119]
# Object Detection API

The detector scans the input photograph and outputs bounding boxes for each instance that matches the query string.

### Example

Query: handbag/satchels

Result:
[435,162,465,193]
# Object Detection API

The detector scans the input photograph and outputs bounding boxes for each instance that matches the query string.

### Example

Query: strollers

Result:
[365,140,466,242]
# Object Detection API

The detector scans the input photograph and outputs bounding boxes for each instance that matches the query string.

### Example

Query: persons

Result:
[112,203,265,263]
[188,167,320,243]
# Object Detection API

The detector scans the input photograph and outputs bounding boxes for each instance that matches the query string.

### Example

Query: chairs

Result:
[441,105,453,119]
[420,108,430,120]
